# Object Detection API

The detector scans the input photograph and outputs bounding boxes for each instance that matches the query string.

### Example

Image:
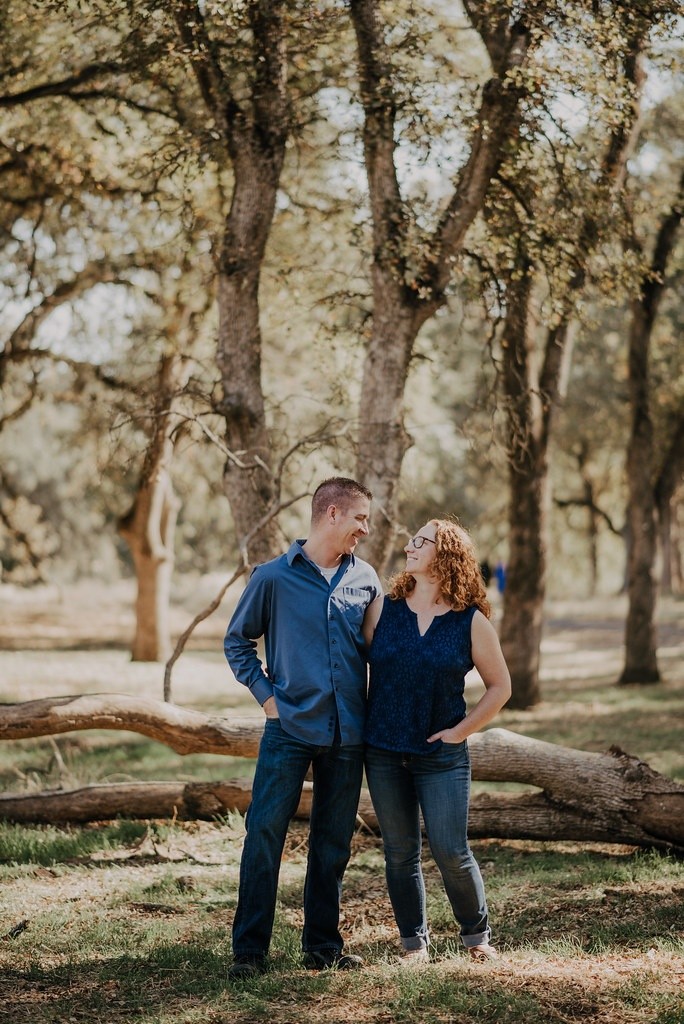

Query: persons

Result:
[224,477,383,978]
[264,519,511,966]
[480,555,505,594]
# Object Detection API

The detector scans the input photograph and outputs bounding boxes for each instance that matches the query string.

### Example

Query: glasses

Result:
[408,536,436,549]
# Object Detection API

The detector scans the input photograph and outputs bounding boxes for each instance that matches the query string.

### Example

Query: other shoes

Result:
[401,949,429,967]
[468,945,498,964]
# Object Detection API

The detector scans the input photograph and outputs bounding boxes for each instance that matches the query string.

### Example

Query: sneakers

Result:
[229,953,266,981]
[304,949,364,970]
